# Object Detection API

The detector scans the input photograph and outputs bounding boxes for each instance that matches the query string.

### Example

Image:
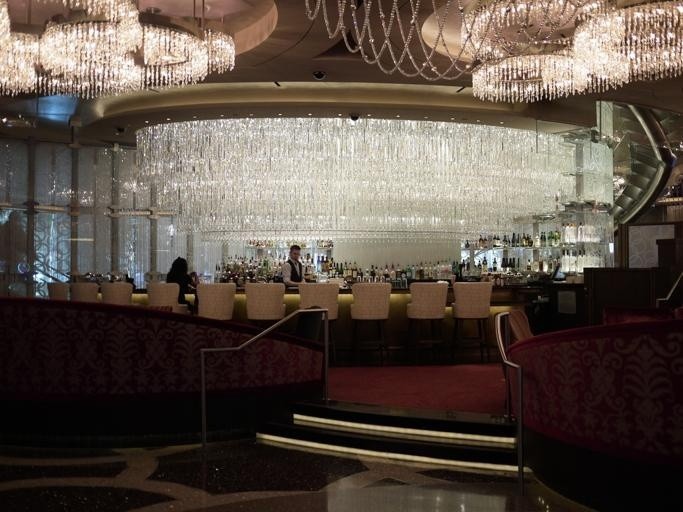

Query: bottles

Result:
[217,231,560,286]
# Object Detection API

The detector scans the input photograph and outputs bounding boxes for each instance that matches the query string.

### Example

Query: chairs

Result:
[245,282,286,331]
[46,281,69,301]
[100,281,133,306]
[350,283,392,361]
[298,282,340,363]
[405,281,448,358]
[70,282,99,303]
[451,281,492,359]
[196,282,236,321]
[146,282,189,314]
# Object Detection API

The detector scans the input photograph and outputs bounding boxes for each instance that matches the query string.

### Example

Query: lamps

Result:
[460,0,682,103]
[0,1,236,99]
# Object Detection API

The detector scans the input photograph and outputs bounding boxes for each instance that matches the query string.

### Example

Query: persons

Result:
[165,255,201,314]
[279,245,309,294]
